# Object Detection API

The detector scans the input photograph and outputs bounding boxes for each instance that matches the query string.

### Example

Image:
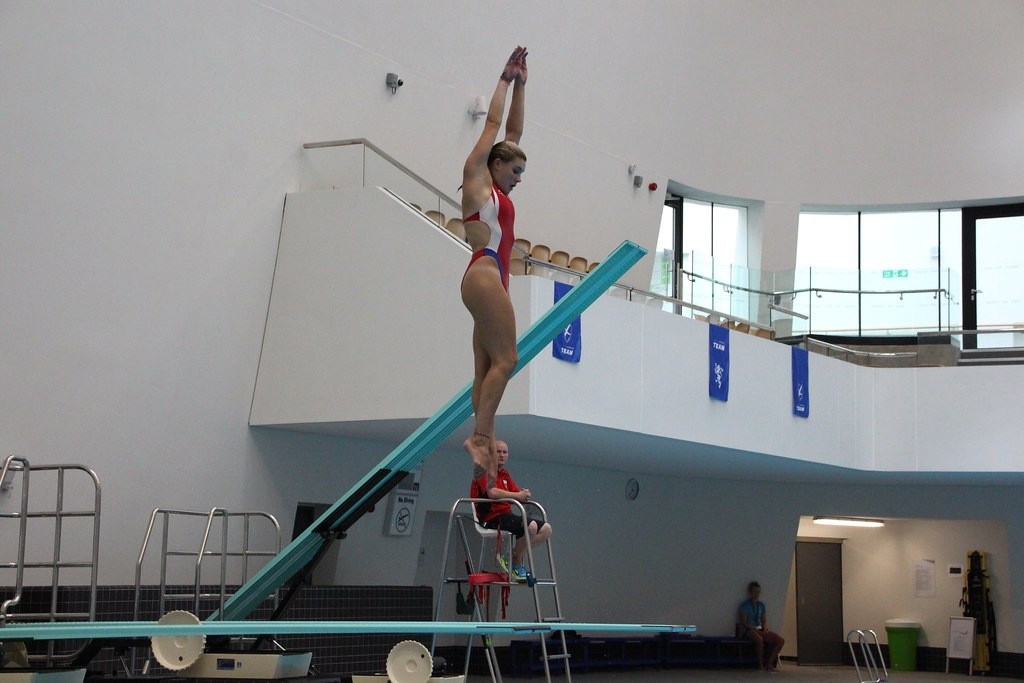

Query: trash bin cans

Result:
[884,618,920,672]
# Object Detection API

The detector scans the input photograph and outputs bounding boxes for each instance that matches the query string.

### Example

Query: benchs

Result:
[509,637,783,681]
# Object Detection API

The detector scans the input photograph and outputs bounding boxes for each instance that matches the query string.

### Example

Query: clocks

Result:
[625,479,639,501]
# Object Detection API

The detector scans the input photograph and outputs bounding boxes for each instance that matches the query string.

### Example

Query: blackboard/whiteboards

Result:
[946,616,975,658]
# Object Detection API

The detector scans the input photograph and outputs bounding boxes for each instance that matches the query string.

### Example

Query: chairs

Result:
[693,314,777,341]
[471,502,513,583]
[409,201,601,276]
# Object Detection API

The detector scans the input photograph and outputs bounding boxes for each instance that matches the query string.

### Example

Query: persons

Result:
[738,582,785,672]
[469,439,552,581]
[457,44,529,492]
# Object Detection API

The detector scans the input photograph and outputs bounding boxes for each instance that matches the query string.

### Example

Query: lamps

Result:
[469,94,487,118]
[813,515,885,527]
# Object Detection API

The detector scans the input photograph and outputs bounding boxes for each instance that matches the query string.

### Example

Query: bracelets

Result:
[499,75,512,85]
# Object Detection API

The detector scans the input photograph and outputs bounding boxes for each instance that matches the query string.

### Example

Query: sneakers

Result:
[496,550,530,580]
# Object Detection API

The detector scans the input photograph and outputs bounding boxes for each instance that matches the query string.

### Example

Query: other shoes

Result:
[766,664,779,672]
